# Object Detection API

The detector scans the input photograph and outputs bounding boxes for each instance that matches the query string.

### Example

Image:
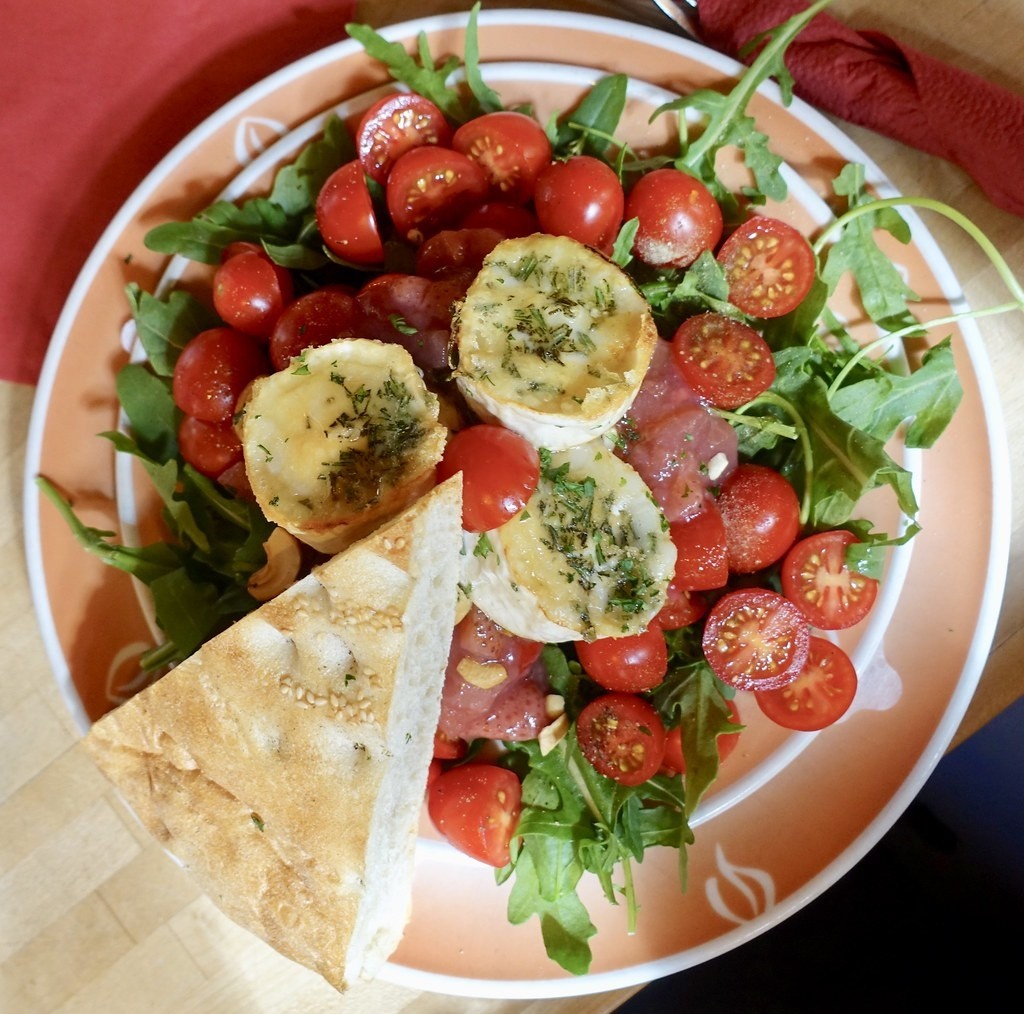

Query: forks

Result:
[653,0,702,43]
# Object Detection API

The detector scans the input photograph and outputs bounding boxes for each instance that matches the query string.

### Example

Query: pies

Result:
[86,472,464,991]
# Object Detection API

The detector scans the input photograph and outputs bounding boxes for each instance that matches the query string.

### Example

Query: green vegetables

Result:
[35,0,1022,984]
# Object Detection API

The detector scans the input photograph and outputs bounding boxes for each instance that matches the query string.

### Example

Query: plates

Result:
[21,8,1013,999]
